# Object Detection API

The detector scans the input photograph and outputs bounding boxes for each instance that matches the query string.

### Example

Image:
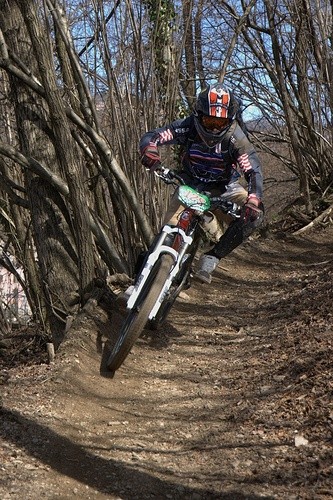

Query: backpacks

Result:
[231,95,249,138]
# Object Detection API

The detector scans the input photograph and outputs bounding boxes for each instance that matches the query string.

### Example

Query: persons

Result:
[124,86,264,298]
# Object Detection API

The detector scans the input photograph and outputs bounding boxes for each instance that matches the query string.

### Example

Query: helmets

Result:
[191,83,239,149]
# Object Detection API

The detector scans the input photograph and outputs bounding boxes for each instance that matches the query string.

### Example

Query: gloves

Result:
[140,142,161,170]
[236,198,265,236]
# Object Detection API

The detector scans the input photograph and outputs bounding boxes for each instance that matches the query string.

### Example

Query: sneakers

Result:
[191,253,220,284]
